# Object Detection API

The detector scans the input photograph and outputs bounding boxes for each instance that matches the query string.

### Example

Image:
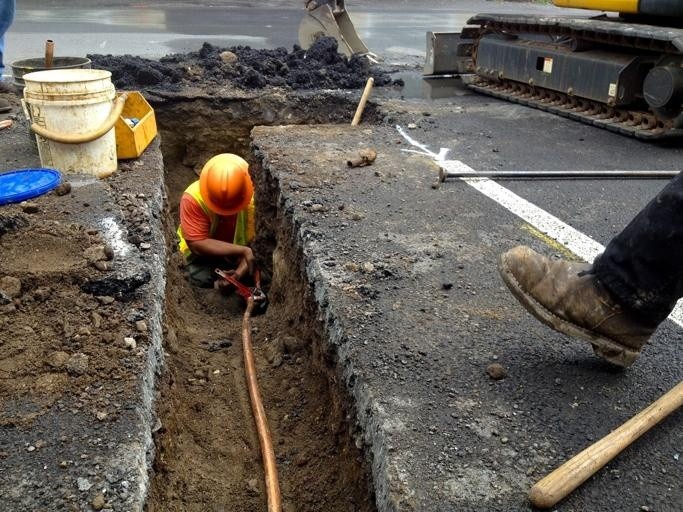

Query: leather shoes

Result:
[497,244,657,369]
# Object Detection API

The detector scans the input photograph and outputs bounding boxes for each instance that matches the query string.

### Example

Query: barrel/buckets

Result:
[21,68,119,179]
[11,57,92,155]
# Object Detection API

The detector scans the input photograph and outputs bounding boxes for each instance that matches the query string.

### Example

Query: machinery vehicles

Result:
[296,0,680,153]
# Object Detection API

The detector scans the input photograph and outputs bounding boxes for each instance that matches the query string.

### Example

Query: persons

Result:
[175,153,257,293]
[494,170,683,367]
[0,0,16,114]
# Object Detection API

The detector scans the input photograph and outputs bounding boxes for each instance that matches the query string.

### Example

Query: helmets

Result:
[200,153,255,216]
